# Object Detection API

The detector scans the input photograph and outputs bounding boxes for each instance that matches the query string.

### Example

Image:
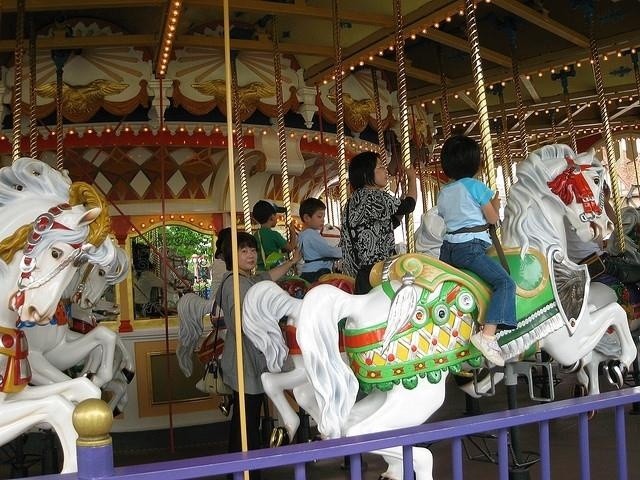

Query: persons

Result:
[563,213,639,286]
[133,243,165,319]
[336,149,418,279]
[297,196,343,284]
[252,200,299,281]
[212,226,239,299]
[435,133,518,368]
[212,229,303,480]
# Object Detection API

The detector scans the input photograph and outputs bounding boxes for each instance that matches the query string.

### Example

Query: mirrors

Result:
[129,220,216,321]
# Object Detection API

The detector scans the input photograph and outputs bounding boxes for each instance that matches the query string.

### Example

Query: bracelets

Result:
[288,258,297,267]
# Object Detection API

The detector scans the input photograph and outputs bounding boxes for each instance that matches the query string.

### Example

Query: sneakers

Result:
[469,330,504,368]
